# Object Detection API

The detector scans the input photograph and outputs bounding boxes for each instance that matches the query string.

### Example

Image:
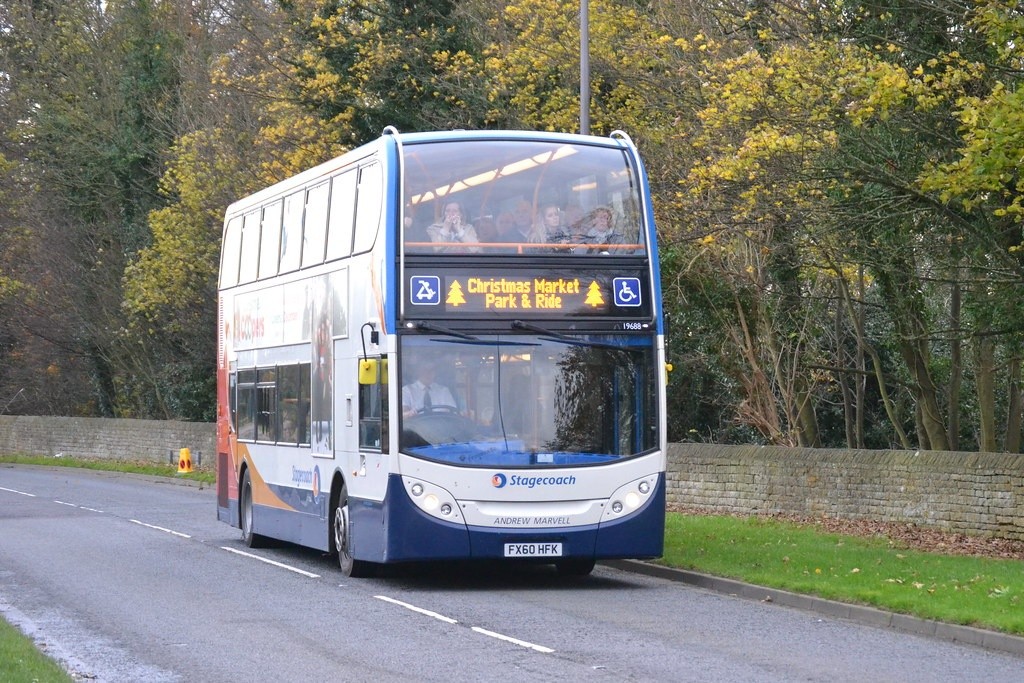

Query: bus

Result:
[213,126,674,578]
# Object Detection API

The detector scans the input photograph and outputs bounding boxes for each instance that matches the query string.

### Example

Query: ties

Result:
[424,386,432,415]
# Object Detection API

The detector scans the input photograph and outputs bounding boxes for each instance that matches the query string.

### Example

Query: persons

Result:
[495,201,532,252]
[527,204,585,254]
[402,361,456,417]
[476,209,513,242]
[283,420,294,442]
[583,208,626,254]
[426,199,479,252]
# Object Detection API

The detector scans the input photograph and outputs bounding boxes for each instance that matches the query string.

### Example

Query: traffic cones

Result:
[177,448,194,474]
